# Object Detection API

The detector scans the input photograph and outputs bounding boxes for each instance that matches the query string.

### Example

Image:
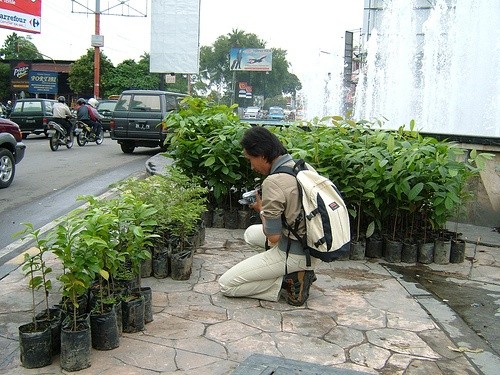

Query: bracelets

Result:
[260,211,263,214]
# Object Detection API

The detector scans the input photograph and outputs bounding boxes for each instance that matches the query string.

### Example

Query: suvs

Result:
[10,98,58,140]
[0,132,27,189]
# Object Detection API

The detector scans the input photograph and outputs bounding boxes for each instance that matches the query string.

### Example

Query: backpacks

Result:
[269,158,352,263]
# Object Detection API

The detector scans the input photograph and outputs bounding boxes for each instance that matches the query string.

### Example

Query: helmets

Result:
[58,96,65,102]
[7,100,12,104]
[76,98,86,105]
[88,98,99,108]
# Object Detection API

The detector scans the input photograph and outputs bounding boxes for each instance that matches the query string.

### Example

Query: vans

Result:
[109,89,191,153]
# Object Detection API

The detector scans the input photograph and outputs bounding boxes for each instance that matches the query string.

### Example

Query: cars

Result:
[243,106,296,121]
[0,118,23,143]
[70,95,122,133]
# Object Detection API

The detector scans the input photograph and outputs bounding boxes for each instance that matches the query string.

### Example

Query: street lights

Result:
[14,42,59,100]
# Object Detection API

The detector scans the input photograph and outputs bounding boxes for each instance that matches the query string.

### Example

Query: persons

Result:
[218,128,319,306]
[52,96,73,140]
[6,101,12,117]
[88,98,104,139]
[76,98,98,138]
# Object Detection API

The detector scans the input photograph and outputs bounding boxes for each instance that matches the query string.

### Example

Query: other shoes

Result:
[285,272,317,306]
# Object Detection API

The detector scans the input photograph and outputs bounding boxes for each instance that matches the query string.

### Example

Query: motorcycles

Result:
[45,115,74,151]
[72,120,105,146]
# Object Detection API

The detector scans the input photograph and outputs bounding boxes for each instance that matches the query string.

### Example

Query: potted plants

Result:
[11,97,495,370]
